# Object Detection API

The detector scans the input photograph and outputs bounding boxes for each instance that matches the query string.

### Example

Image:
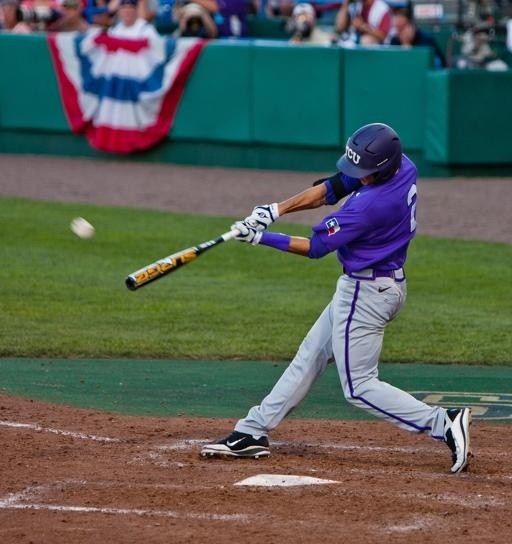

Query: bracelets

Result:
[259,231,290,251]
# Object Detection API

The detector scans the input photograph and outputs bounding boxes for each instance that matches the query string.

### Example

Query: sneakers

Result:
[198,428,272,460]
[441,403,473,474]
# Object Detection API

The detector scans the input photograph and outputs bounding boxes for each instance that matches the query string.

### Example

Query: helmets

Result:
[334,121,404,184]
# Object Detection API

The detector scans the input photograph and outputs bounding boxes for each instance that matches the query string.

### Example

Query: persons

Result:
[201,124,473,475]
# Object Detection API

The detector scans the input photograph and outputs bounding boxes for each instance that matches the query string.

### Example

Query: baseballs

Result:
[70,218,93,238]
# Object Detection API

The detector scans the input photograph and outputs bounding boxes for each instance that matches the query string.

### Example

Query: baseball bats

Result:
[125,229,239,290]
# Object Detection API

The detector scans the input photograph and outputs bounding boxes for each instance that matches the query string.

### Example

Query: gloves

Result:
[229,218,264,247]
[243,201,282,231]
